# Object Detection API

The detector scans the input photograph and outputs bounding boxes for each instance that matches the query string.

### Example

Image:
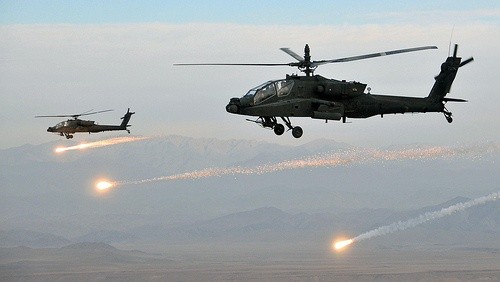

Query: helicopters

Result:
[35,109,136,139]
[171,43,474,138]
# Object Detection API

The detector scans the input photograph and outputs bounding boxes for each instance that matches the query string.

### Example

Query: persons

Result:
[278,82,288,95]
